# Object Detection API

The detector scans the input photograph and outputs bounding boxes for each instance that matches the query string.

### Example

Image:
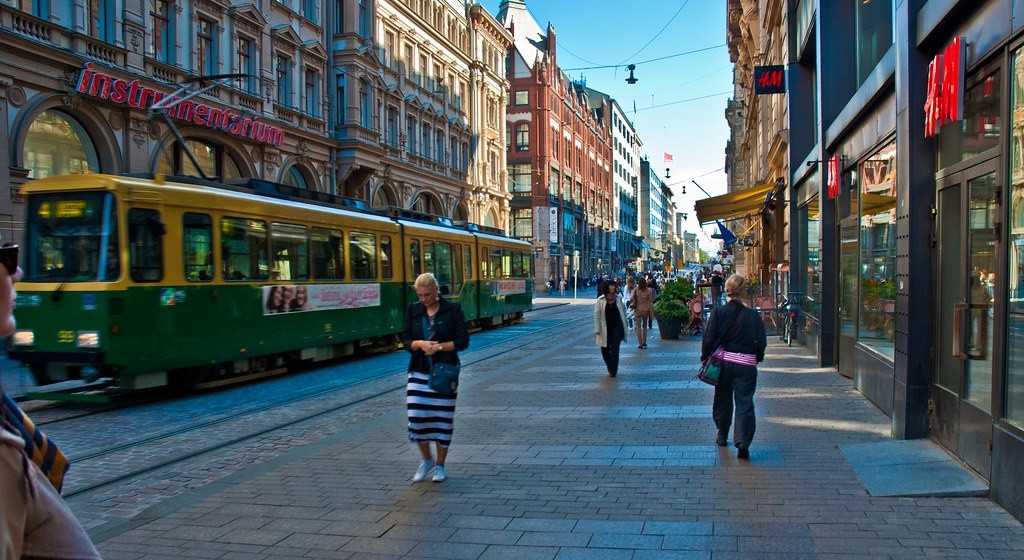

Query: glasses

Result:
[0,243,19,275]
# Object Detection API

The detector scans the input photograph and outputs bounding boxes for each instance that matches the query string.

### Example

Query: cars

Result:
[630,268,701,285]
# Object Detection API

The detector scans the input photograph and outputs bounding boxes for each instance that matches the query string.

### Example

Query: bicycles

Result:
[775,292,809,347]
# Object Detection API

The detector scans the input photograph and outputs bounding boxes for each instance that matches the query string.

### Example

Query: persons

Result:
[0,235,102,560]
[597,268,726,349]
[400,272,469,483]
[263,286,315,314]
[546,275,596,296]
[594,279,628,377]
[700,274,767,459]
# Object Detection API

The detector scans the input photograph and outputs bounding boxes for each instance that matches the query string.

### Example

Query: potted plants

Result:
[653,296,692,339]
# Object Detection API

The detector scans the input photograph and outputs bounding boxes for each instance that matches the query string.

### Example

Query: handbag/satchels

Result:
[698,345,724,386]
[564,282,566,287]
[629,289,637,308]
[429,363,459,395]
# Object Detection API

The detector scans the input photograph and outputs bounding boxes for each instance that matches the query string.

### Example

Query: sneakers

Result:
[414,457,435,482]
[428,465,446,482]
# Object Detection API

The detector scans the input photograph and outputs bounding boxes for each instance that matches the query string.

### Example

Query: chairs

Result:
[742,294,776,332]
[863,296,895,341]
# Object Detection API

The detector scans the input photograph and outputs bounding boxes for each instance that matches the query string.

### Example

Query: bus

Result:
[4,74,537,403]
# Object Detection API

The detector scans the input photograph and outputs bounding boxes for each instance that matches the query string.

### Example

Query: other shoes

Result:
[716,434,728,446]
[737,443,749,459]
[643,343,648,348]
[629,327,632,330]
[638,345,642,348]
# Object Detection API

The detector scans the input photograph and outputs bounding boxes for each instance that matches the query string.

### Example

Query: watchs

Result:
[437,344,443,351]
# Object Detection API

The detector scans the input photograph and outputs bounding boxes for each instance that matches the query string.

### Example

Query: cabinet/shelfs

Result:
[696,283,721,324]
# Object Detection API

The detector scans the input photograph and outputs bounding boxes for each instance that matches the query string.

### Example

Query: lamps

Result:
[765,188,777,204]
[769,201,775,210]
[737,64,753,119]
[625,65,638,84]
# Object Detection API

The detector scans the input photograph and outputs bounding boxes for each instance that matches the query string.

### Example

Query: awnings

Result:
[694,182,780,228]
